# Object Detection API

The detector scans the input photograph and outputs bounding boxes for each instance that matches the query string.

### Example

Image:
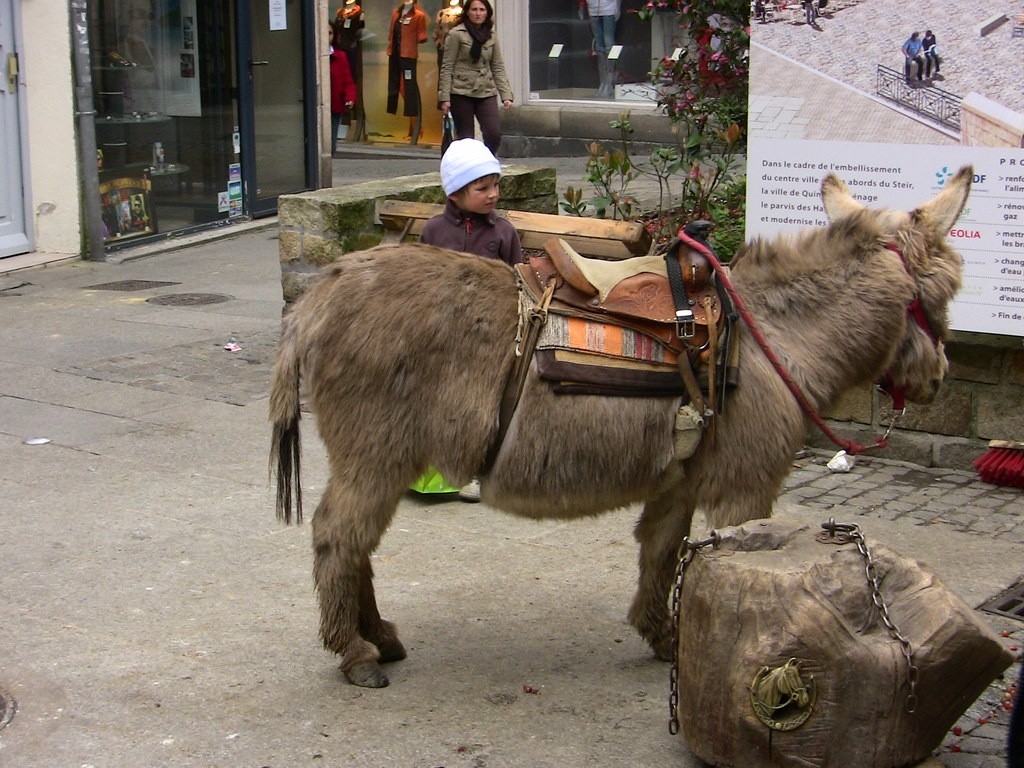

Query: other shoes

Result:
[459,475,481,502]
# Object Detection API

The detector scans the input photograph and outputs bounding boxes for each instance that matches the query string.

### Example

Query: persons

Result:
[335,0,364,125]
[435,0,464,73]
[329,21,357,157]
[438,0,514,155]
[752,0,940,80]
[385,0,429,118]
[418,139,523,500]
[578,0,620,99]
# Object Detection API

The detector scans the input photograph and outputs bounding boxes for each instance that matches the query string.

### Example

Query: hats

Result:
[440,138,503,197]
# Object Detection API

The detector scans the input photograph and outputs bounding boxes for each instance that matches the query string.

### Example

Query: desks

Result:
[142,162,194,218]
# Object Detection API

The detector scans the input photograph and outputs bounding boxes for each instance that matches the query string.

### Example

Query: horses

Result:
[264,161,976,690]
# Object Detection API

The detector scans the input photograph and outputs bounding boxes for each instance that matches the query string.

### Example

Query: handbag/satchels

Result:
[440,110,455,160]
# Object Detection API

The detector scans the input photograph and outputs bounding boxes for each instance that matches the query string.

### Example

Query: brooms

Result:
[972,439,1024,489]
